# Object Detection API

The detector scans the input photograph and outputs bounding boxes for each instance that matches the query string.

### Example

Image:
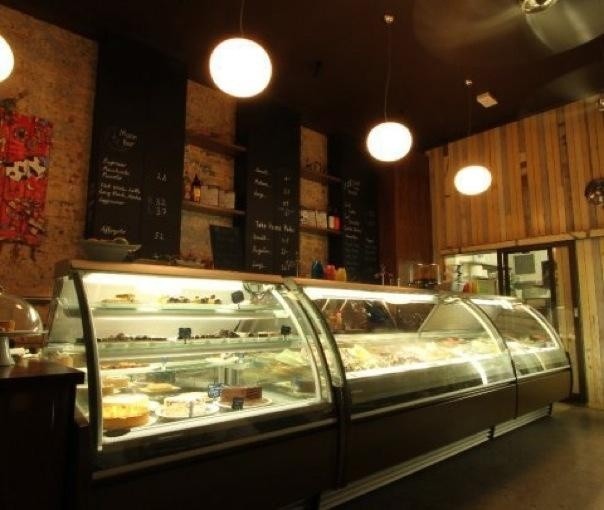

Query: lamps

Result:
[208,1,275,100]
[365,15,414,164]
[585,178,604,210]
[453,80,492,197]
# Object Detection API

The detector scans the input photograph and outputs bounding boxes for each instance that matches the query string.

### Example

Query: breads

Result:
[0,320,14,331]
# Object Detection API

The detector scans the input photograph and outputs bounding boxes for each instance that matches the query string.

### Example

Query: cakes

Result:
[220,385,262,405]
[103,375,129,388]
[102,392,149,419]
[158,295,222,305]
[147,382,172,392]
[101,291,143,306]
[164,391,218,417]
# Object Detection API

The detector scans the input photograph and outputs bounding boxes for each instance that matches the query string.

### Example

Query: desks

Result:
[0,355,84,510]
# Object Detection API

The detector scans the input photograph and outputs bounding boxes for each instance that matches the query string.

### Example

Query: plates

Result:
[88,302,267,313]
[69,240,141,263]
[103,387,274,430]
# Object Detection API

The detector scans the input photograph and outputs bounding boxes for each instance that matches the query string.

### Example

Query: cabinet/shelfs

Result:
[225,274,517,482]
[418,291,573,439]
[47,257,339,510]
[296,167,345,279]
[179,129,247,270]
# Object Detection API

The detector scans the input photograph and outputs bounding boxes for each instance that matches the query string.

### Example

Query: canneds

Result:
[325,265,336,279]
[336,267,346,280]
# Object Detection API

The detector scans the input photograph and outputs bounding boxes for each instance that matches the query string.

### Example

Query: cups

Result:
[414,264,437,290]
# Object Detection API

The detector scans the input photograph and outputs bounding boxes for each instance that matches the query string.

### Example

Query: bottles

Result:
[184,170,201,202]
